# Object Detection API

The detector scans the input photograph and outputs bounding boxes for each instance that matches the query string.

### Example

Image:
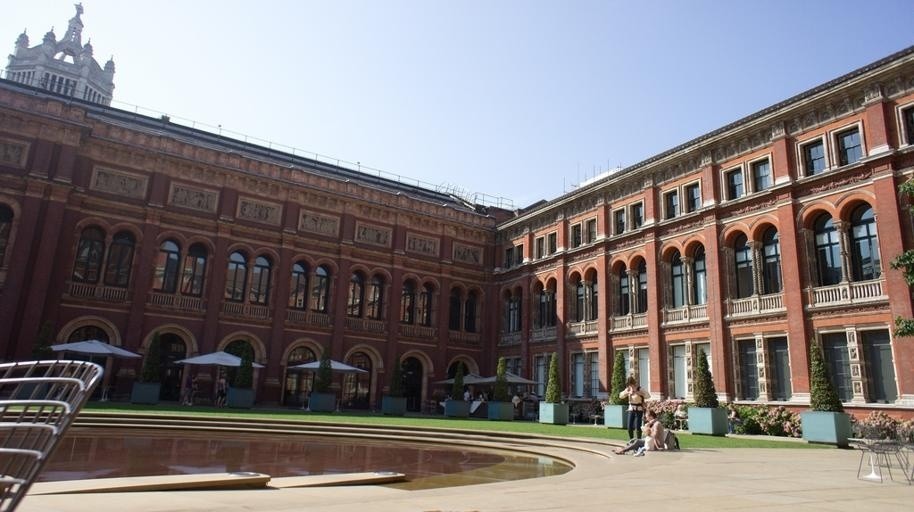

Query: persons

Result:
[187,372,199,404]
[443,387,537,421]
[611,408,664,456]
[619,376,650,439]
[178,370,192,405]
[727,404,739,434]
[216,372,226,408]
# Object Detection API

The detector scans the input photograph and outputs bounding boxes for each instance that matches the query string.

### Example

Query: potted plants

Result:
[683,345,731,438]
[129,330,164,406]
[796,336,855,447]
[485,356,515,422]
[600,351,635,431]
[307,337,338,414]
[380,354,410,418]
[537,351,572,427]
[225,337,257,411]
[440,360,471,420]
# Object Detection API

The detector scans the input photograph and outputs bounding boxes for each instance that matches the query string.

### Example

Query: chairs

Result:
[846,424,913,485]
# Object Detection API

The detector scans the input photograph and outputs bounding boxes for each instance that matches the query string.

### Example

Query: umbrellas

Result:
[172,350,265,404]
[431,372,484,385]
[474,371,544,387]
[286,357,370,376]
[50,338,142,364]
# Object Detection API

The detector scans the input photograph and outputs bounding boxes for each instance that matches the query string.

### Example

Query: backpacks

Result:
[665,431,680,450]
